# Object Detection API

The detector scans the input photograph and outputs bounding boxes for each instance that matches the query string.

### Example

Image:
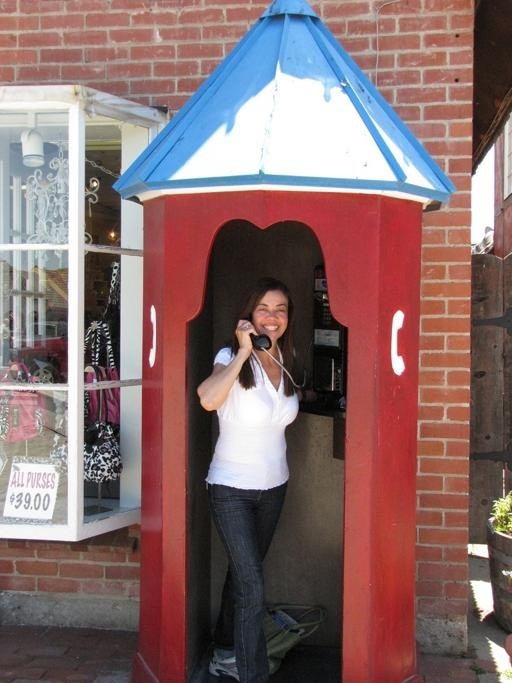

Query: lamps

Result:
[19,113,45,166]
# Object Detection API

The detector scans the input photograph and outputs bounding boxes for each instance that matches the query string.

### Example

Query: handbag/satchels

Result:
[0,362,120,483]
[262,605,327,674]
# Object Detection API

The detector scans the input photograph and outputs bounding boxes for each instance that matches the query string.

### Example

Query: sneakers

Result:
[209,649,240,682]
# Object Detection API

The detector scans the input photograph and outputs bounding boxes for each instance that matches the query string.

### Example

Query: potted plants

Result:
[485,488,511,633]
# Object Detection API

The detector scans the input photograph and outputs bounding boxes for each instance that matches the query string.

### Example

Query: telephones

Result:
[237,312,272,351]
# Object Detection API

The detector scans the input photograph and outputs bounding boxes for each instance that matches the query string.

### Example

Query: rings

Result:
[246,324,249,329]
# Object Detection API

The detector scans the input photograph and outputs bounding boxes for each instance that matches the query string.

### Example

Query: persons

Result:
[196,276,301,683]
